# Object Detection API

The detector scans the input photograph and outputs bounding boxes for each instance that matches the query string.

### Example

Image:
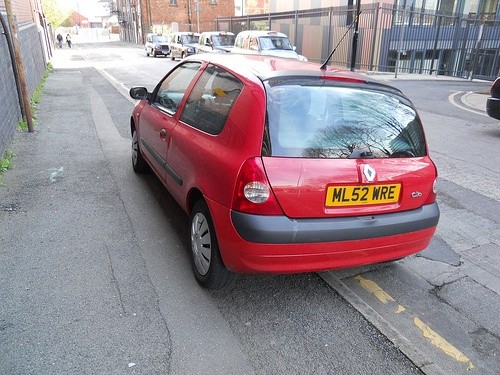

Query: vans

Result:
[233,31,308,65]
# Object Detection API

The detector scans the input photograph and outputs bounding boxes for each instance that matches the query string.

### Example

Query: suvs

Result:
[144,32,171,58]
[169,32,200,60]
[195,31,235,53]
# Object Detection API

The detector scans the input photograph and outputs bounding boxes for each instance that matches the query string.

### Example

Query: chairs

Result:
[204,71,243,117]
[280,85,318,148]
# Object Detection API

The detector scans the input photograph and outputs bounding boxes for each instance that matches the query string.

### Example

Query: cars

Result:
[127,54,440,287]
[486,77,500,121]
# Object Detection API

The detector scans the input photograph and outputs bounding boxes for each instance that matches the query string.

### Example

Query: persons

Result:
[66,34,72,48]
[57,33,63,48]
